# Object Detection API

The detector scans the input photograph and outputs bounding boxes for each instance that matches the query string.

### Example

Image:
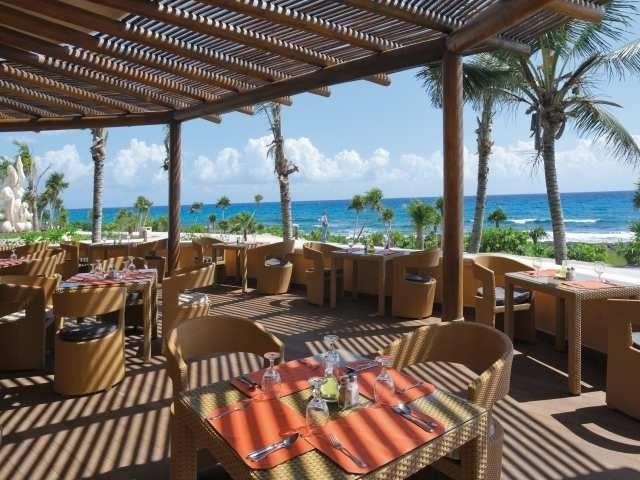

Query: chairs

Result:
[465,252,639,418]
[192,237,441,321]
[163,259,216,350]
[0,241,164,399]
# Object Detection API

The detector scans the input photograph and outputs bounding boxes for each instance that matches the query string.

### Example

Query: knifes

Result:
[392,407,433,434]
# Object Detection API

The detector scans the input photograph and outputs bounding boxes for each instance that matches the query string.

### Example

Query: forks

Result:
[326,433,367,468]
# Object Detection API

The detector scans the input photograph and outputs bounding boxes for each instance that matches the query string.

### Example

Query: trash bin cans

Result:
[140,227,152,238]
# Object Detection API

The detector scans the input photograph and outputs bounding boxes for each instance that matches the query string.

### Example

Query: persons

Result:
[320,211,330,243]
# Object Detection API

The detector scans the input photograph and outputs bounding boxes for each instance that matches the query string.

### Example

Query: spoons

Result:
[398,404,438,428]
[252,433,300,463]
[238,375,260,393]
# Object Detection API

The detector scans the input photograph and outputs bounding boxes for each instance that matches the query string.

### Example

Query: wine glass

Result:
[348,239,354,253]
[305,377,329,428]
[261,352,281,391]
[320,335,343,378]
[374,354,394,403]
[533,258,543,279]
[595,261,605,283]
[90,259,104,281]
[122,256,136,273]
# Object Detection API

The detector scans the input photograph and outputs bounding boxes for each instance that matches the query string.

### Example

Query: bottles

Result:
[369,239,374,252]
[348,373,359,407]
[78,258,89,272]
[338,377,352,409]
[565,267,575,280]
